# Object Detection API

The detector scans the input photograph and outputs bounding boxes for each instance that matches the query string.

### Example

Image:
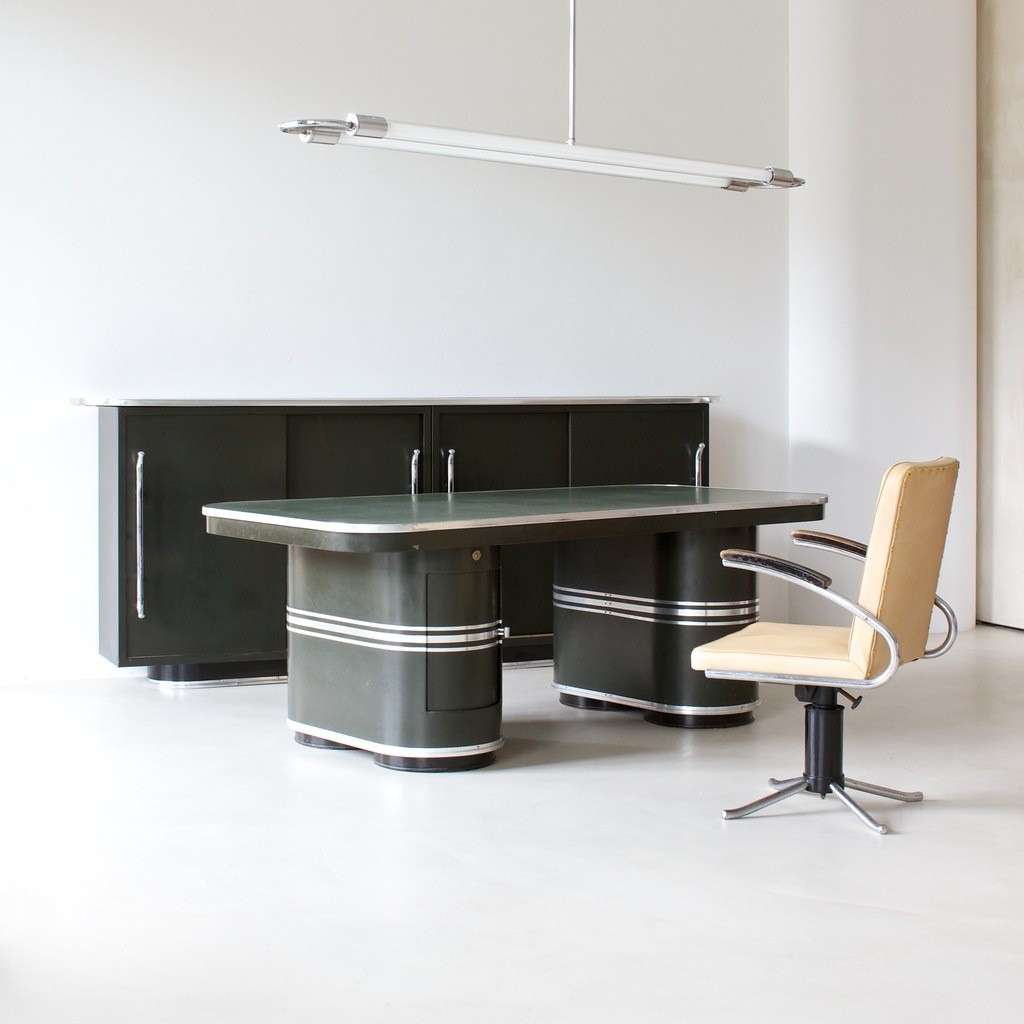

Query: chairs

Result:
[692,457,958,835]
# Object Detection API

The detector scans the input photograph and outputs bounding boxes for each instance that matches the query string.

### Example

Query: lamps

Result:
[283,0,806,192]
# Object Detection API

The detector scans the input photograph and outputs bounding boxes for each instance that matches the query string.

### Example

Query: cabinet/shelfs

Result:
[84,394,721,683]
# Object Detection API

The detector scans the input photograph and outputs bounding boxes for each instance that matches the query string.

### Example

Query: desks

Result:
[201,483,827,772]
